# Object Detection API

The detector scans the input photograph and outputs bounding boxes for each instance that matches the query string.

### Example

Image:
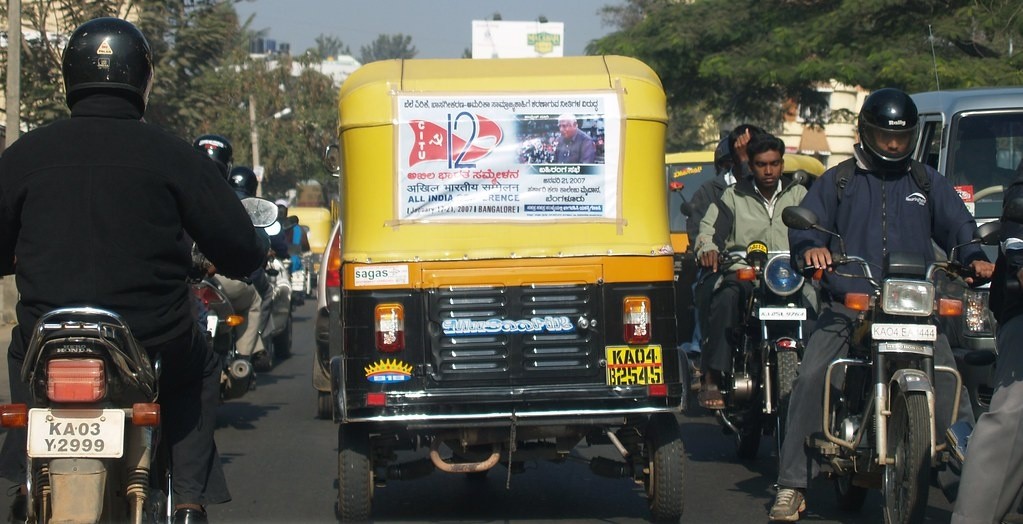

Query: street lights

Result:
[247,94,294,198]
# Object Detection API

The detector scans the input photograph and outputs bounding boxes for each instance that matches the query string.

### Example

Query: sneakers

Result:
[768,483,806,521]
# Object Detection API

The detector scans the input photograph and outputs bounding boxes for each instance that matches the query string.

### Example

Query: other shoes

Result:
[691,365,701,389]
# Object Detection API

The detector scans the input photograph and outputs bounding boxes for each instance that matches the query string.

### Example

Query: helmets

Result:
[228,167,258,197]
[192,134,233,181]
[857,88,920,172]
[61,17,153,116]
[714,137,731,174]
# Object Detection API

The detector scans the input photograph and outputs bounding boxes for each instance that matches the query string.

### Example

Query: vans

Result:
[909,86,1023,427]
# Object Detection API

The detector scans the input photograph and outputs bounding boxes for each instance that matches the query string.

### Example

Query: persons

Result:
[689,123,767,378]
[953,178,1023,523]
[769,88,994,521]
[518,113,605,163]
[185,134,309,359]
[954,130,1017,188]
[0,17,269,524]
[695,134,808,409]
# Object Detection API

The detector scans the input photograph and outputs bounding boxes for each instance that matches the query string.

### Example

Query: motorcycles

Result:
[670,179,987,524]
[189,214,313,405]
[0,196,278,524]
[663,151,828,338]
[337,57,686,524]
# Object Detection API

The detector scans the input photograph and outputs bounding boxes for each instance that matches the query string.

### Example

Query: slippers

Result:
[697,388,726,410]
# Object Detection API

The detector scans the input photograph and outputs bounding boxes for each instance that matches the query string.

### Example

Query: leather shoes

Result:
[12,494,40,524]
[171,504,208,524]
